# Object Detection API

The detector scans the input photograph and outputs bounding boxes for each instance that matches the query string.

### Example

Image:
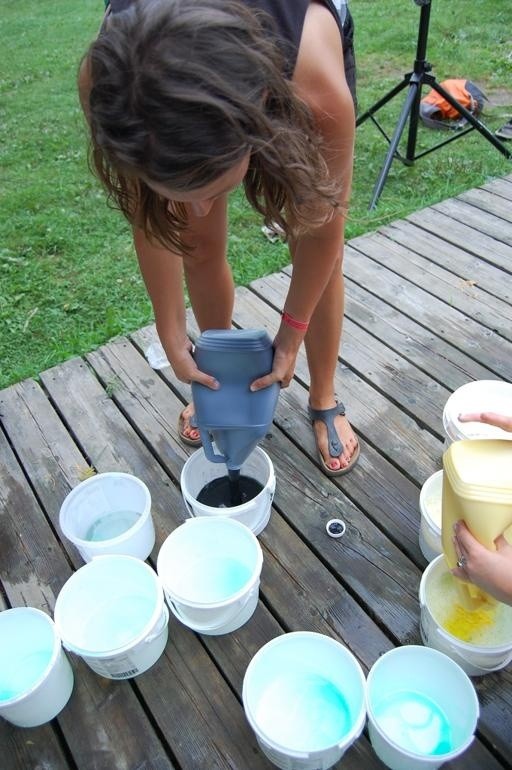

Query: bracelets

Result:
[281,311,311,331]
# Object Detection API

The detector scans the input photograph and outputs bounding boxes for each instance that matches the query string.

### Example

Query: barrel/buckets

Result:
[59,473,155,562]
[53,554,170,681]
[241,631,367,770]
[190,329,281,479]
[443,379,511,455]
[418,470,444,561]
[367,646,480,770]
[442,439,511,581]
[180,439,276,536]
[156,516,264,636]
[418,553,512,677]
[0,607,75,728]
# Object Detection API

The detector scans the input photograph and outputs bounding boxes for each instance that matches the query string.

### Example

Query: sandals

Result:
[178,402,212,444]
[308,395,360,476]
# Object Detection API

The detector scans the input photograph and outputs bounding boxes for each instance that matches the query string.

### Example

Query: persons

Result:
[77,1,361,477]
[448,409,511,607]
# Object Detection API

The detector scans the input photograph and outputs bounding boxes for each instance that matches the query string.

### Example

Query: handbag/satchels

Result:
[419,79,483,129]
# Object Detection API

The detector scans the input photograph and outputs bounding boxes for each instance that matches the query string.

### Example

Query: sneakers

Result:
[495,119,512,138]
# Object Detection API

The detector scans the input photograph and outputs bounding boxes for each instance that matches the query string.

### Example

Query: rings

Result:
[456,556,465,569]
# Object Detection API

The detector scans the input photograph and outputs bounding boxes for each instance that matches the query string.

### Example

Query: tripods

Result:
[356,0,512,211]
